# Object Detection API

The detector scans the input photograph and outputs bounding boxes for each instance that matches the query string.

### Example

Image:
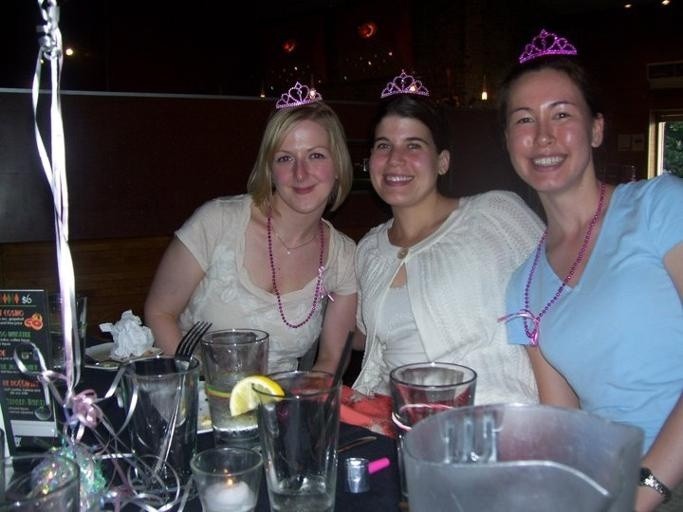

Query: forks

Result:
[149,321,212,490]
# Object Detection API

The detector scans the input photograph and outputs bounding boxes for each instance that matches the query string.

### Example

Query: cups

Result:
[390,362,478,499]
[0,454,79,512]
[201,328,269,448]
[252,371,340,512]
[189,447,263,512]
[121,355,197,480]
[50,294,88,371]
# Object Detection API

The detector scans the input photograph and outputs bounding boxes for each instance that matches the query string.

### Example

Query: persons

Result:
[500,28,682,511]
[347,70,544,441]
[141,80,360,376]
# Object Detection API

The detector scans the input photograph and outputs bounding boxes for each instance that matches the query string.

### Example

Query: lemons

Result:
[229,374,283,418]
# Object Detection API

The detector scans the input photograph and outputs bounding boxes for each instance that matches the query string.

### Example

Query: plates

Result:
[196,379,215,435]
[85,342,163,371]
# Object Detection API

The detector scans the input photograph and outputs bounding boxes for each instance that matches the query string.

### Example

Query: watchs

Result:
[636,467,672,503]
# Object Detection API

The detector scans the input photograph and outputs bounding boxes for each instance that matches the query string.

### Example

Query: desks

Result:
[0,332,682,511]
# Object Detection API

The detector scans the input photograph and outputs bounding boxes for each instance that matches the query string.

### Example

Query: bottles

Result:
[343,457,369,493]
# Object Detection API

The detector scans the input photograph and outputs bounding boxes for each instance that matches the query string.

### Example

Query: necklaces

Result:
[266,202,325,330]
[271,223,319,255]
[523,177,606,340]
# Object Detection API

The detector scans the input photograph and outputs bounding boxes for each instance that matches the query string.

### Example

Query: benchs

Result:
[1,230,172,331]
